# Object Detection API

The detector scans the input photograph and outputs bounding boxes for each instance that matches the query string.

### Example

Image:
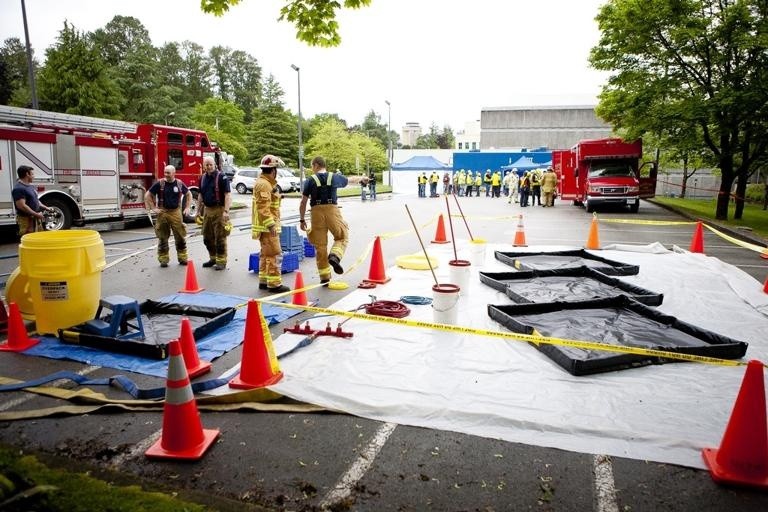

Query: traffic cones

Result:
[430,213,451,243]
[142,340,221,463]
[362,236,394,285]
[581,216,605,250]
[700,357,767,487]
[226,299,285,390]
[289,271,311,306]
[177,259,205,295]
[687,220,704,252]
[0,299,40,353]
[511,214,529,247]
[175,318,213,381]
[762,275,768,295]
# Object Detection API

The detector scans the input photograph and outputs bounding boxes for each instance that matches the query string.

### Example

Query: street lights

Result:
[165,111,175,126]
[383,98,391,185]
[290,62,303,191]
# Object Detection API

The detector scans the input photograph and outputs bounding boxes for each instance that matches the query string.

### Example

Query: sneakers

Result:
[267,283,290,293]
[320,279,330,288]
[203,259,216,268]
[328,256,343,274]
[508,201,555,207]
[258,283,267,289]
[160,263,168,267]
[179,260,188,265]
[215,264,226,270]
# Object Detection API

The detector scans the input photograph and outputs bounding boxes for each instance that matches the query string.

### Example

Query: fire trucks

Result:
[0,104,222,233]
[550,135,657,213]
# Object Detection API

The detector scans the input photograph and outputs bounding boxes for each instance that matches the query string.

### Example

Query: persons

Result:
[193,156,233,272]
[251,153,290,294]
[360,173,371,201]
[368,171,378,201]
[299,156,349,288]
[142,164,194,269]
[416,166,559,207]
[10,165,55,245]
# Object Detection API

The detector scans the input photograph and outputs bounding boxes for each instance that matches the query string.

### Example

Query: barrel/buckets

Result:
[449,258,472,282]
[469,240,488,264]
[431,283,461,320]
[17,229,106,337]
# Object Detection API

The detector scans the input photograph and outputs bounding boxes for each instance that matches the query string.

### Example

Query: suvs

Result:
[223,163,305,194]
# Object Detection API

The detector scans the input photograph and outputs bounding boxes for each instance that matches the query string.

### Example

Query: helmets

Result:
[258,154,286,169]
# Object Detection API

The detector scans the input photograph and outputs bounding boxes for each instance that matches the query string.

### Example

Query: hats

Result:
[429,165,554,177]
